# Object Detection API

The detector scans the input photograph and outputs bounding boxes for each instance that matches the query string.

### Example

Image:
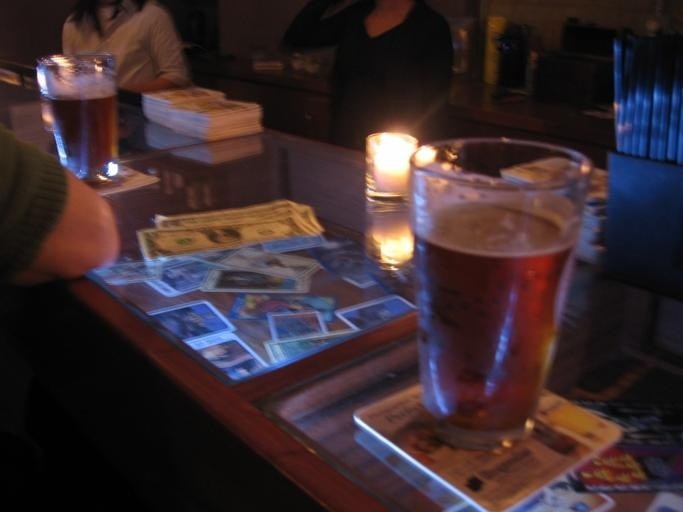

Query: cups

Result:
[363,130,416,199]
[34,50,123,188]
[405,136,596,453]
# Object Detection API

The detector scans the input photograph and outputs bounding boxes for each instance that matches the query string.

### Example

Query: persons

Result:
[0,126,120,288]
[271,1,454,152]
[62,2,191,94]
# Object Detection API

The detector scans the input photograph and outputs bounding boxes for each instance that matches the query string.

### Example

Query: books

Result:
[139,86,264,139]
